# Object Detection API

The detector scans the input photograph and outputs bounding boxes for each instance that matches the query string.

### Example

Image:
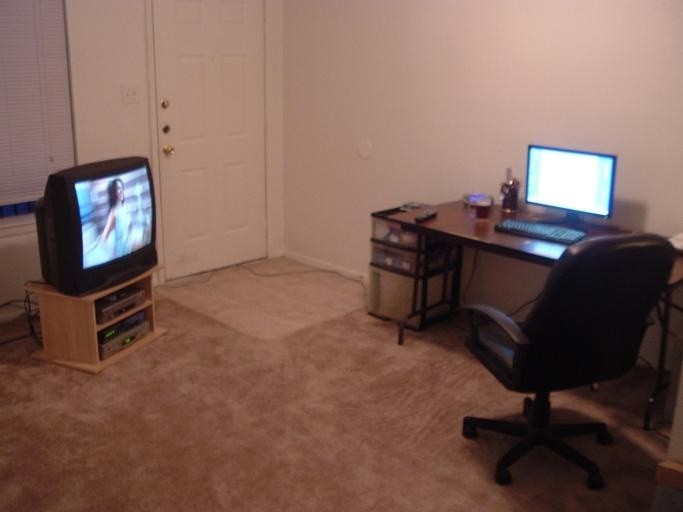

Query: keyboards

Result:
[495,219,586,244]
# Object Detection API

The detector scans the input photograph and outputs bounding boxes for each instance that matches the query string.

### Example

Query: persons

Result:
[100,178,132,258]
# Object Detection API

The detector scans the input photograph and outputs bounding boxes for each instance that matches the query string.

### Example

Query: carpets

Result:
[153,256,367,343]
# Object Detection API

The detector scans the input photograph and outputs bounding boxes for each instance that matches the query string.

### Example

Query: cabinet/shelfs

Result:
[366,200,451,333]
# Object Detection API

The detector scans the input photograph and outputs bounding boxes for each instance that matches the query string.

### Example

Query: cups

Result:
[469,199,491,224]
[501,188,520,213]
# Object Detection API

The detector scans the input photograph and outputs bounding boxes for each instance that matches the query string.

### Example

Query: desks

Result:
[24,260,167,373]
[388,195,683,432]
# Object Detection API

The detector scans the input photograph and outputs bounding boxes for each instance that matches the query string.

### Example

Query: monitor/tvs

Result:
[35,157,158,297]
[525,144,617,232]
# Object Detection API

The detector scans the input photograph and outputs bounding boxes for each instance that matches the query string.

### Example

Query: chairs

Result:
[462,232,678,490]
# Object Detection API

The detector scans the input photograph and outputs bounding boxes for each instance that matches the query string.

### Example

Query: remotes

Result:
[415,207,437,222]
